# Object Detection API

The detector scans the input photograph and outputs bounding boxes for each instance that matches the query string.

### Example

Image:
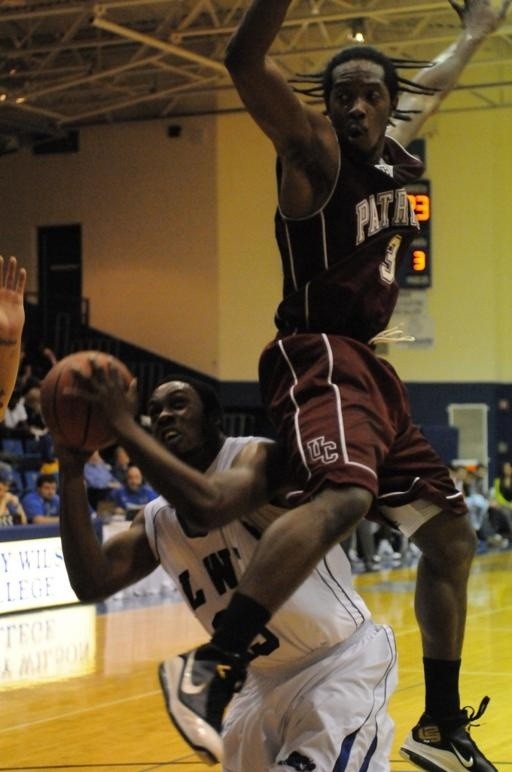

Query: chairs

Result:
[1,439,44,498]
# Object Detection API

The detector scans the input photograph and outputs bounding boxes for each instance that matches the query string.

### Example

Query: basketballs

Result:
[42,351,137,452]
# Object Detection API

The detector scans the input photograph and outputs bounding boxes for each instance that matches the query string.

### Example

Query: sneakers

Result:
[400,696,499,772]
[157,643,247,765]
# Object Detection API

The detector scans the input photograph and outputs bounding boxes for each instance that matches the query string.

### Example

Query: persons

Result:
[0,254,29,434]
[43,361,399,771]
[338,455,512,578]
[0,339,161,530]
[152,1,512,772]
